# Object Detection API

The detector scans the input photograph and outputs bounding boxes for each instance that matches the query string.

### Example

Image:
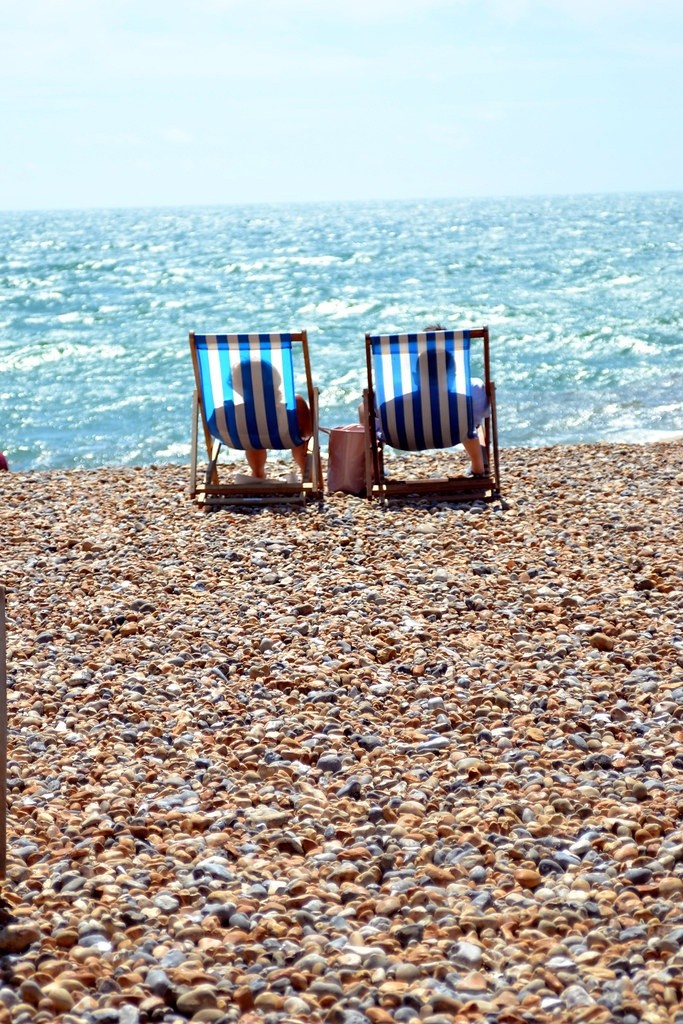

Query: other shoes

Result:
[465,464,486,477]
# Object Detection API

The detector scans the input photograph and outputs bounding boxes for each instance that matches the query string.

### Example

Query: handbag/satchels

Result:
[319,421,374,498]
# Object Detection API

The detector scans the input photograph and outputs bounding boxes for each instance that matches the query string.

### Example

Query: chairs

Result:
[190,329,327,507]
[364,326,500,499]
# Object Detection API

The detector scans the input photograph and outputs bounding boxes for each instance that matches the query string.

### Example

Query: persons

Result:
[207,360,313,478]
[357,324,487,476]
